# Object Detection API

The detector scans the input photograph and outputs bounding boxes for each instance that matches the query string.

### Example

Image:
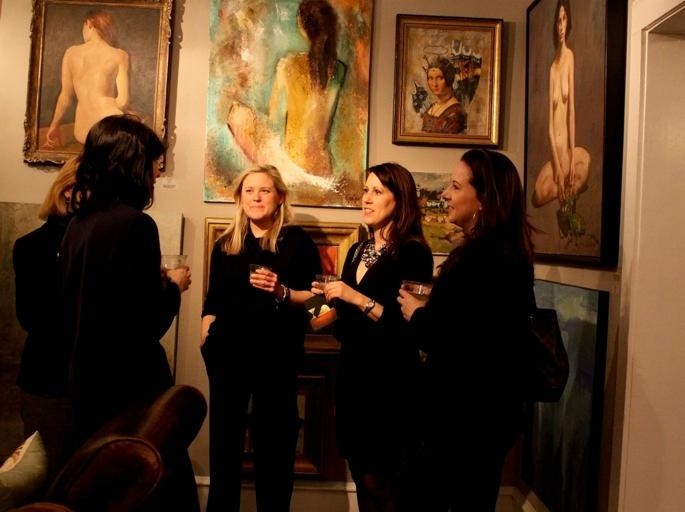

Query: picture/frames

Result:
[523,0,628,273]
[239,374,350,483]
[20,0,172,175]
[391,12,503,148]
[202,214,362,356]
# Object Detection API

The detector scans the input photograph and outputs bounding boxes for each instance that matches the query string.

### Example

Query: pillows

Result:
[0,428,49,511]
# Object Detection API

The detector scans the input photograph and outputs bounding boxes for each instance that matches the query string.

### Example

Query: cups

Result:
[316,272,339,289]
[402,279,433,302]
[249,266,274,284]
[161,253,189,275]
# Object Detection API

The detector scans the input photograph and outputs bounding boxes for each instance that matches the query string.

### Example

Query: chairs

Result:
[1,383,208,512]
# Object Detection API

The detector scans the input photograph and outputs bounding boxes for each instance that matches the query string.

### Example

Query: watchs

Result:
[364,299,375,314]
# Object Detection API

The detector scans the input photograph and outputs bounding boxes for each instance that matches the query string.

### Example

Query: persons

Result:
[59,115,201,512]
[311,162,433,512]
[228,0,347,192]
[394,150,536,512]
[13,155,92,482]
[532,0,591,208]
[199,165,322,512]
[41,10,153,149]
[421,59,467,134]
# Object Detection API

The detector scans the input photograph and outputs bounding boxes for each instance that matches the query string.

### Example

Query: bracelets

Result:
[275,284,289,304]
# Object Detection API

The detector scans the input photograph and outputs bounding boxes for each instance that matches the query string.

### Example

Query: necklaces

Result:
[360,238,394,268]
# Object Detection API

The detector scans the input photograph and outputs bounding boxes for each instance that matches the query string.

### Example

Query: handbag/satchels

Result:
[533,309,568,402]
